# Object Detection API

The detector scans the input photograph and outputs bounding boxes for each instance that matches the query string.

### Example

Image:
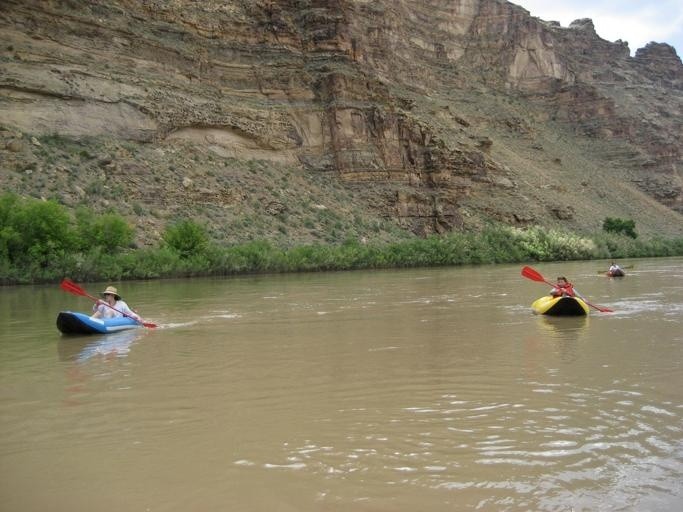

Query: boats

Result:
[532,296,589,314]
[56,309,144,334]
[607,270,625,276]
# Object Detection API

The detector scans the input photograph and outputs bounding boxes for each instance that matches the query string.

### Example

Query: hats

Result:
[99,286,121,301]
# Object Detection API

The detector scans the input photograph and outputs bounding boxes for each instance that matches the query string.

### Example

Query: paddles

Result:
[58,278,158,328]
[598,265,635,275]
[520,265,613,313]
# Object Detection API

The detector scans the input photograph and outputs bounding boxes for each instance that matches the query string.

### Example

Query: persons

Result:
[91,286,142,323]
[609,262,621,270]
[550,277,586,302]
